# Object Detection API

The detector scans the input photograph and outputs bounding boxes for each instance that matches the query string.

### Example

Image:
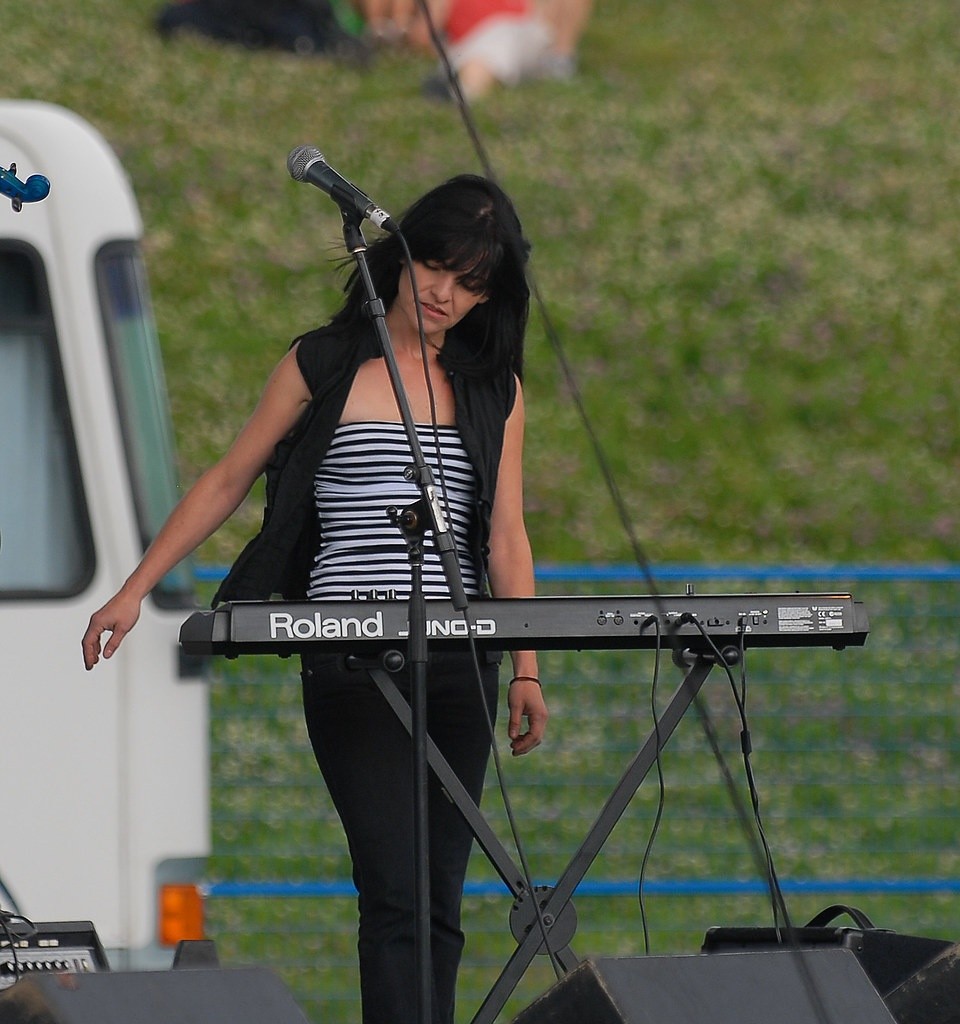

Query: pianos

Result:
[175,590,876,661]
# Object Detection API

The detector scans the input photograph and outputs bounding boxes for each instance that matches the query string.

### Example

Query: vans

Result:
[1,97,218,981]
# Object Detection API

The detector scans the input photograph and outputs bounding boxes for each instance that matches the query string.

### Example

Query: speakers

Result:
[1,964,311,1023]
[510,925,960,1024]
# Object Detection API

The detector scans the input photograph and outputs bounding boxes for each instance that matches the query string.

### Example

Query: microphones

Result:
[284,147,400,237]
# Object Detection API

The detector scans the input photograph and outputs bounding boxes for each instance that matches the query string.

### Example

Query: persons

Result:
[80,175,549,1024]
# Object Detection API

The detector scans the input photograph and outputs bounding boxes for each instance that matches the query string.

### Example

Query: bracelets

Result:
[510,676,542,688]
